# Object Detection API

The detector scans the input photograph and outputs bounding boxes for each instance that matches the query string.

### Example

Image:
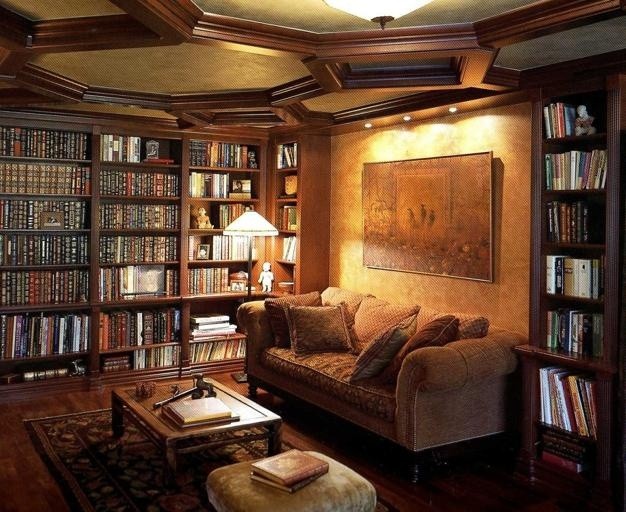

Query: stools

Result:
[207,448,380,512]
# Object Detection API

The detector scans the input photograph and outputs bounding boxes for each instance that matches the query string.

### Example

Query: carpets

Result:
[22,404,395,512]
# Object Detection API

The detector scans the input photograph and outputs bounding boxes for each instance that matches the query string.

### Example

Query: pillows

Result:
[282,299,353,353]
[264,290,325,349]
[348,311,417,381]
[379,314,458,386]
[458,313,490,342]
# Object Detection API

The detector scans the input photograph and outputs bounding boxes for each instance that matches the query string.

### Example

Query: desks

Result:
[110,372,284,493]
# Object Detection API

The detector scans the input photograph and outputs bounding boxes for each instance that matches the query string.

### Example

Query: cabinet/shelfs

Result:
[519,57,625,512]
[0,108,97,391]
[185,131,271,376]
[273,134,334,294]
[96,119,185,386]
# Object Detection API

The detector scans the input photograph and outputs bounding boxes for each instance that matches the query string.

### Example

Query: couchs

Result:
[232,283,525,491]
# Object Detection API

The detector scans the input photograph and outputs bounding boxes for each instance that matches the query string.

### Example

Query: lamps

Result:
[221,204,278,382]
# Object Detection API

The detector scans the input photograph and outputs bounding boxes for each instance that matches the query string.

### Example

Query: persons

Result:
[200,247,207,257]
[234,282,242,290]
[428,208,435,229]
[194,207,214,229]
[363,222,490,280]
[258,261,274,293]
[407,208,415,236]
[421,204,427,226]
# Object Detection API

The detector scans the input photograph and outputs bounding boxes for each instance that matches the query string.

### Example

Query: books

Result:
[248,469,328,494]
[536,102,610,475]
[166,396,232,424]
[1,126,90,229]
[204,235,256,260]
[161,405,240,428]
[250,447,329,485]
[1,313,91,359]
[190,141,247,169]
[277,142,297,263]
[99,201,178,263]
[188,266,228,295]
[210,203,254,229]
[99,265,177,303]
[100,307,182,372]
[189,171,251,199]
[18,361,69,382]
[100,134,140,163]
[1,232,88,306]
[100,167,179,197]
[188,236,194,261]
[189,313,247,362]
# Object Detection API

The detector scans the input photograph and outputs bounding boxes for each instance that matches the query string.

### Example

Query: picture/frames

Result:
[195,243,210,261]
[362,151,495,283]
[39,210,64,231]
[144,138,159,160]
